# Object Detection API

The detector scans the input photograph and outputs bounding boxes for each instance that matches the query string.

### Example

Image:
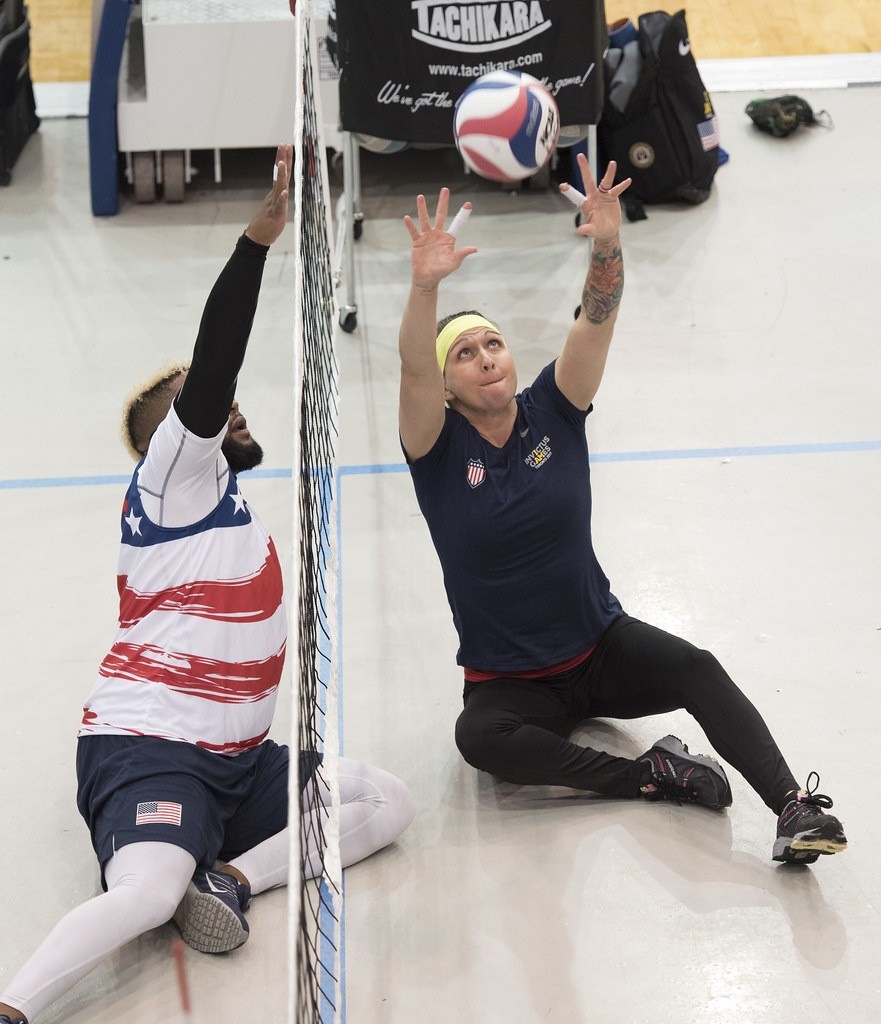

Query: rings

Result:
[598,184,612,194]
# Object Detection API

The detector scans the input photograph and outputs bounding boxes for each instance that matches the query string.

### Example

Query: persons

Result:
[396,151,851,866]
[0,142,417,1024]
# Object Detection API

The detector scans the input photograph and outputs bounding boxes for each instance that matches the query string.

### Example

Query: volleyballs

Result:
[450,69,562,184]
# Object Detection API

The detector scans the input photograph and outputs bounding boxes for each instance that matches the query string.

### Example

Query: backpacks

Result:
[598,9,719,223]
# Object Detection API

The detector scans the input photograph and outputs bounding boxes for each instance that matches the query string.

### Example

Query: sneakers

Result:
[168,865,250,953]
[634,734,732,810]
[772,772,848,864]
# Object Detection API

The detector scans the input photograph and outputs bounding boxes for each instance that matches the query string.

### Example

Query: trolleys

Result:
[321,1,608,336]
